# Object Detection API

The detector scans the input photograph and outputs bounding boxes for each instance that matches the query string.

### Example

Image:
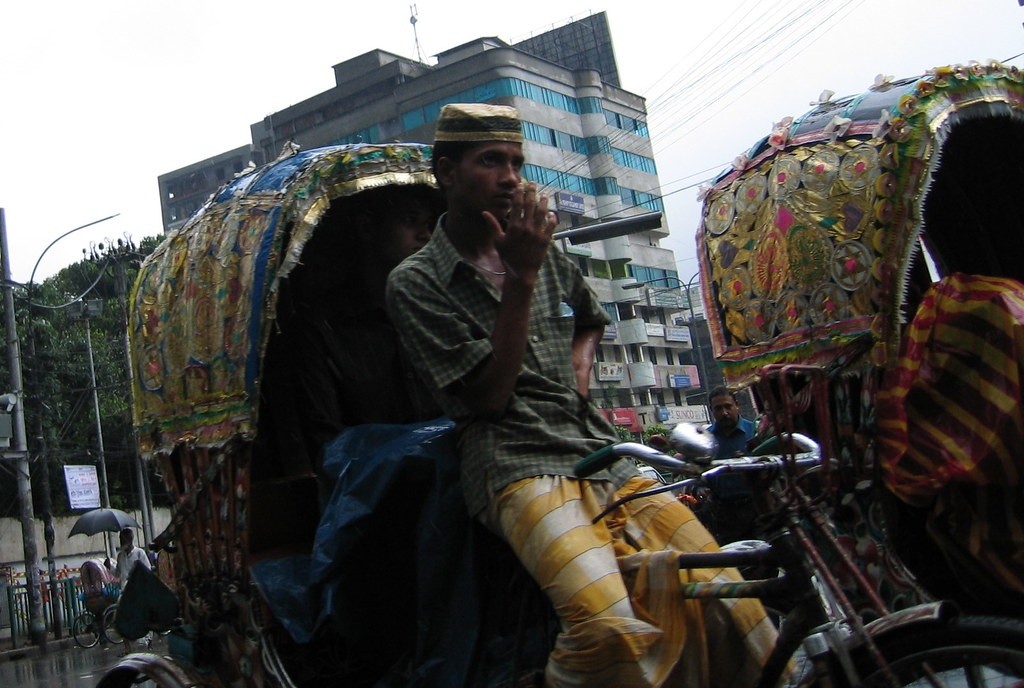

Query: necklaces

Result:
[463,258,507,277]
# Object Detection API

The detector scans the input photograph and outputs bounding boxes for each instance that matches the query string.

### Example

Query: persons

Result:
[690,383,761,546]
[294,183,554,687]
[105,528,151,657]
[382,101,808,686]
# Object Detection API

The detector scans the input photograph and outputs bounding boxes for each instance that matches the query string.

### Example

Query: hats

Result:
[434,102,523,143]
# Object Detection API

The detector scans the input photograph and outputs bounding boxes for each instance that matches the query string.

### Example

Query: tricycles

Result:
[92,138,1024,688]
[71,550,172,649]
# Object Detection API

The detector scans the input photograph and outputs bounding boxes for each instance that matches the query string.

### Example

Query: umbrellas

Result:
[66,505,142,565]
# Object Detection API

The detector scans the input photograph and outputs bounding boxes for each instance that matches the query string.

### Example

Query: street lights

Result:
[620,271,716,426]
[68,298,118,560]
